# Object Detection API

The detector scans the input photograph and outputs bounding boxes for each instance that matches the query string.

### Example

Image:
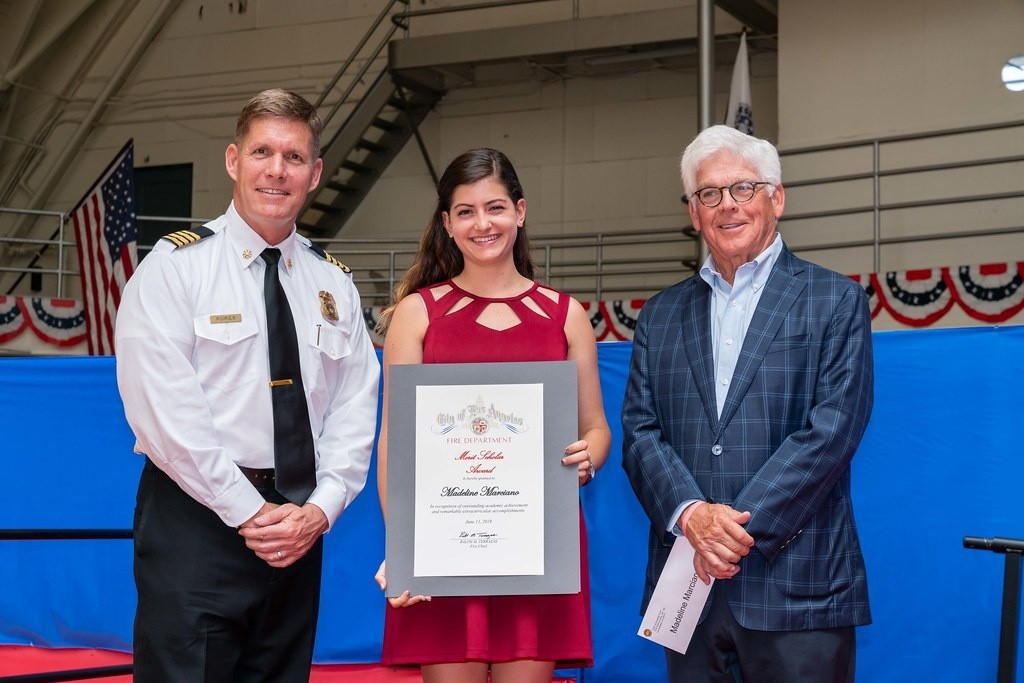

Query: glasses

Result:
[691,180,772,207]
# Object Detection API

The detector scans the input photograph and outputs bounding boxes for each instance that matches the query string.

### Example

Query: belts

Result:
[240,466,275,487]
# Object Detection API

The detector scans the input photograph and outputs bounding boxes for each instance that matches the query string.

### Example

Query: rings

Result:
[277,551,283,561]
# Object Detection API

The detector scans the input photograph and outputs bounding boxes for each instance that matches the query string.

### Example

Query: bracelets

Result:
[681,500,708,538]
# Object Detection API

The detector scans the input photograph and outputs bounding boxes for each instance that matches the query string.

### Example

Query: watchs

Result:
[584,459,595,486]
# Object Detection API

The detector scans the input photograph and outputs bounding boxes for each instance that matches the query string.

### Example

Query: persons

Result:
[619,123,876,682]
[114,84,381,683]
[372,147,613,683]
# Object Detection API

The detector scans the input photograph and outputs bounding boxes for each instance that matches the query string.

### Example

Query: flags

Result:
[71,143,138,356]
[725,31,753,137]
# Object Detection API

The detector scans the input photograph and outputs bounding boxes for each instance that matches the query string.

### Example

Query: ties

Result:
[260,248,317,507]
